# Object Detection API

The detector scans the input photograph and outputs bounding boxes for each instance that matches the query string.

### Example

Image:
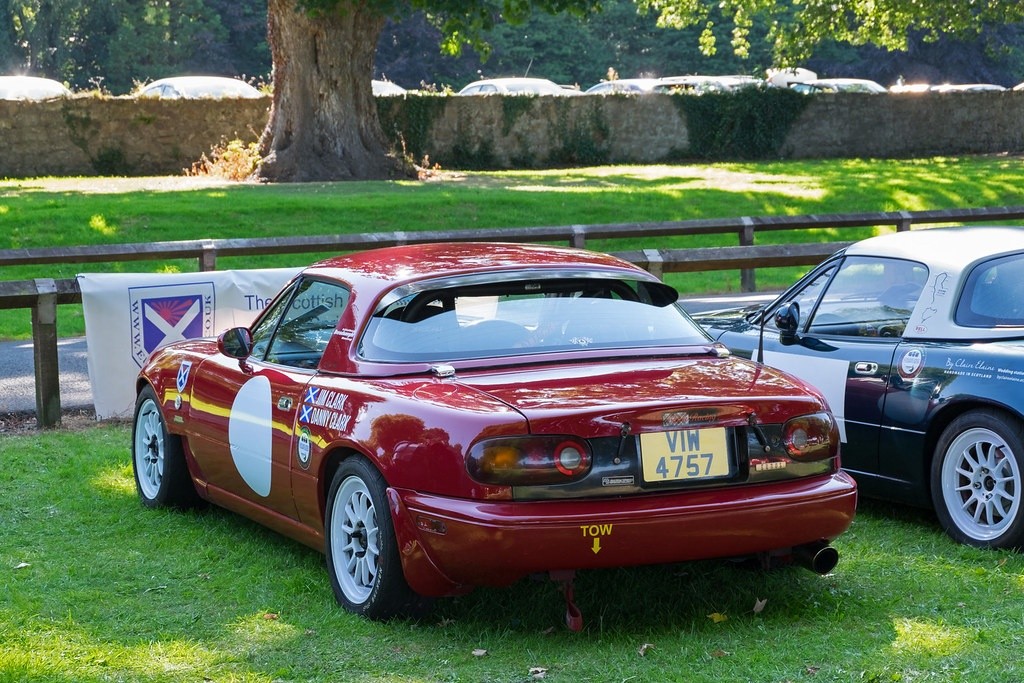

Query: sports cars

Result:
[650,227,1024,553]
[132,243,859,620]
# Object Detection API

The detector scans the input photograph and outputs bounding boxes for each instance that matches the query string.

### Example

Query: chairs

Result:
[381,306,460,352]
